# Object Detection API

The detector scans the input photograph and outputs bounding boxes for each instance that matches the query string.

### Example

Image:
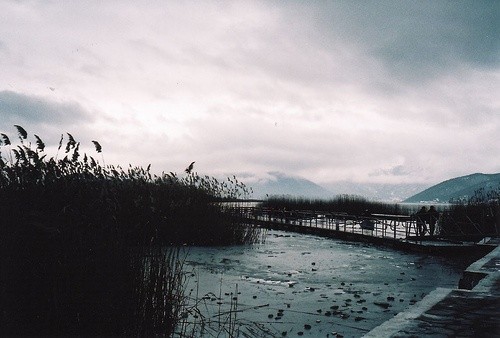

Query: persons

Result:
[425,206,439,236]
[364,209,371,222]
[415,207,428,237]
